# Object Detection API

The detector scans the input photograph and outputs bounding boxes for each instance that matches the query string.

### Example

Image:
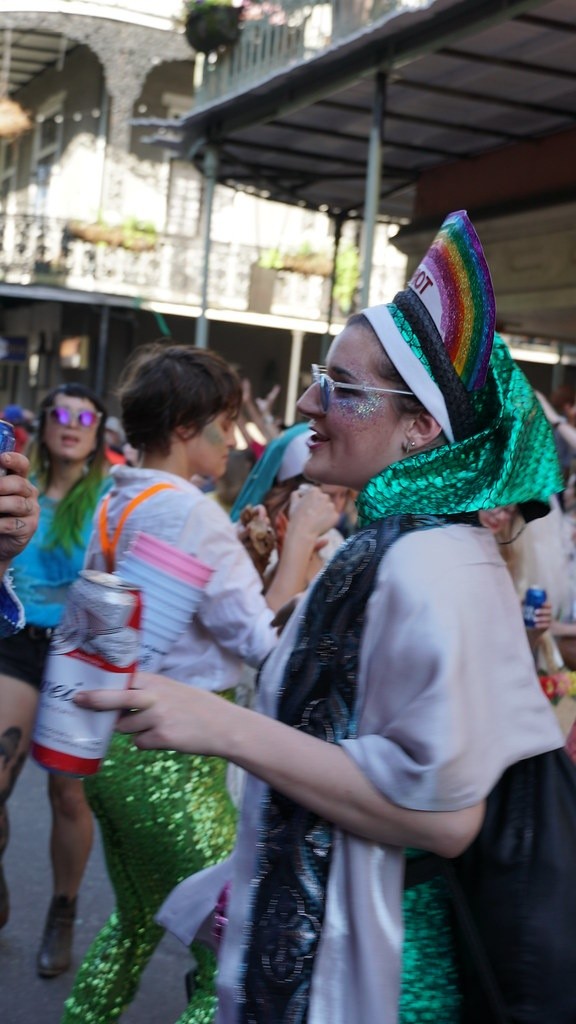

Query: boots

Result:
[38,900,77,976]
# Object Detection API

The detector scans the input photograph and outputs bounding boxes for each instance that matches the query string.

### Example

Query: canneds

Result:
[0,419,16,477]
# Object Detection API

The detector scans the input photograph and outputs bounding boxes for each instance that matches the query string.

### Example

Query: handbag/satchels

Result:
[439,745,576,1024]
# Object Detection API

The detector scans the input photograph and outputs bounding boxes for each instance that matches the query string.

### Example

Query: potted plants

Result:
[66,220,175,290]
[246,247,329,321]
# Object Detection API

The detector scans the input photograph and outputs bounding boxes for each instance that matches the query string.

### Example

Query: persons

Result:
[478,473,576,671]
[3,405,29,453]
[59,346,340,1024]
[102,417,132,467]
[0,382,113,974]
[205,376,359,589]
[73,291,576,1024]
[0,452,41,639]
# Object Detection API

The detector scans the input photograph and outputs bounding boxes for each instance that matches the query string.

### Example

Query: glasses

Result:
[47,407,102,429]
[310,363,415,413]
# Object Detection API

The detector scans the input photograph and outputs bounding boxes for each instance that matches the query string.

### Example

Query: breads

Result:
[32,569,143,777]
[522,585,547,626]
